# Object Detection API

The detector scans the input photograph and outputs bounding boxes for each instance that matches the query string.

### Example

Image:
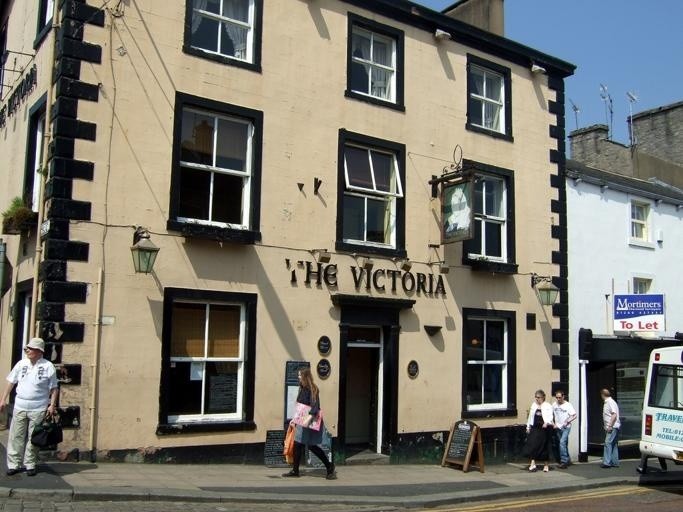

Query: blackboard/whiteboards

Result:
[209,373,236,412]
[444,420,478,465]
[264,430,294,468]
[305,433,332,467]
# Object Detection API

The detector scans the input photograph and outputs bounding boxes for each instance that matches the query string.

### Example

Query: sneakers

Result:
[543,465,549,472]
[528,465,537,472]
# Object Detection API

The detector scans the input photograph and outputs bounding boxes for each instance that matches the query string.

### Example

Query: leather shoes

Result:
[600,464,610,469]
[27,468,38,476]
[7,468,23,476]
[282,470,300,477]
[326,462,335,479]
[555,463,568,470]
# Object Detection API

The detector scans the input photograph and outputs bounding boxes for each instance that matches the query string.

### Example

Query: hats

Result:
[26,337,45,353]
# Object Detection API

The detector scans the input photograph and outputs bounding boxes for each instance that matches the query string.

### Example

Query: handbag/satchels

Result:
[30,412,63,451]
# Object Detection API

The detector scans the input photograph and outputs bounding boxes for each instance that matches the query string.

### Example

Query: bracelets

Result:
[50,401,56,405]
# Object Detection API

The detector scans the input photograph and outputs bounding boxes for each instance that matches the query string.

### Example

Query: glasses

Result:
[556,396,562,399]
[534,396,543,398]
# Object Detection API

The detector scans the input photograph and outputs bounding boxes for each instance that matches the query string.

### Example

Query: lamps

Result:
[131,226,162,273]
[531,274,560,305]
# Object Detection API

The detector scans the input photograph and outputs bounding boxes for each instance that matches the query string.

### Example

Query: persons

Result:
[0,338,58,476]
[281,366,335,479]
[522,389,555,472]
[599,388,621,468]
[549,391,578,468]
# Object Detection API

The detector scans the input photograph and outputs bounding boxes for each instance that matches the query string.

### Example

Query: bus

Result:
[638,344,683,464]
[616,367,647,432]
